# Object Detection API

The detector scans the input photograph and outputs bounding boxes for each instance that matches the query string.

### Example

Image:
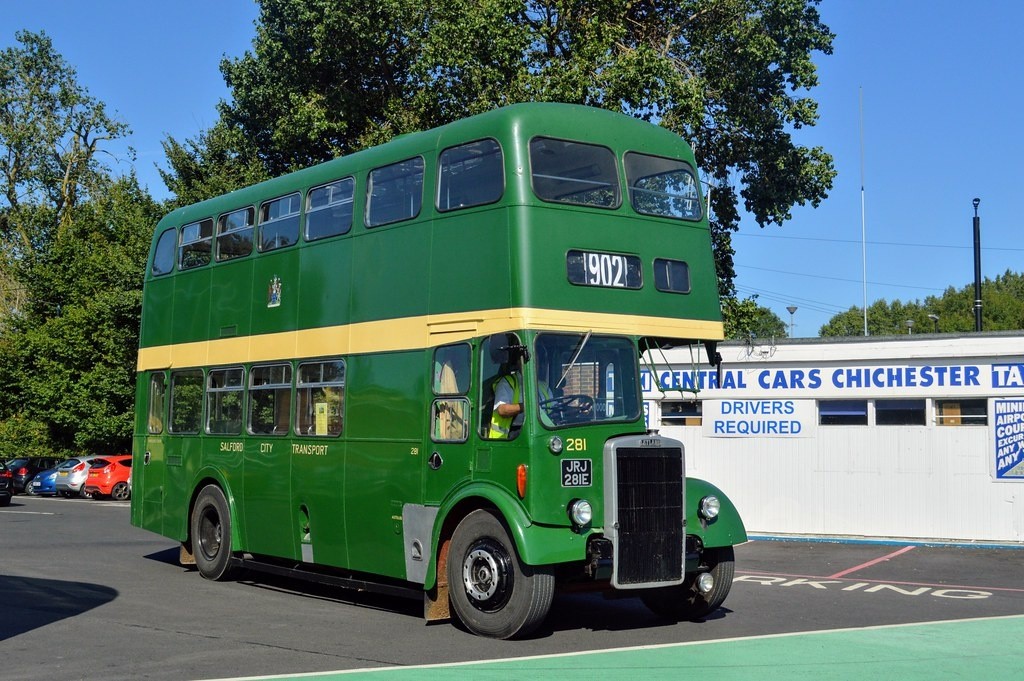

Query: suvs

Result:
[7,456,67,497]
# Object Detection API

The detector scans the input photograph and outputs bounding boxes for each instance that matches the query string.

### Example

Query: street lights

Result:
[786,306,798,338]
[905,319,914,335]
[927,315,939,332]
[972,197,982,332]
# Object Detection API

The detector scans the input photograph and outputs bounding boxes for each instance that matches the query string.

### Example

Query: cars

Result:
[54,455,103,499]
[33,465,59,498]
[85,454,133,500]
[0,460,13,506]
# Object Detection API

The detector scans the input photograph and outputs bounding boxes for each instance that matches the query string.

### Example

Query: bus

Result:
[131,101,749,641]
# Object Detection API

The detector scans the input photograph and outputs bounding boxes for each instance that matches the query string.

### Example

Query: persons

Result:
[490,350,593,441]
